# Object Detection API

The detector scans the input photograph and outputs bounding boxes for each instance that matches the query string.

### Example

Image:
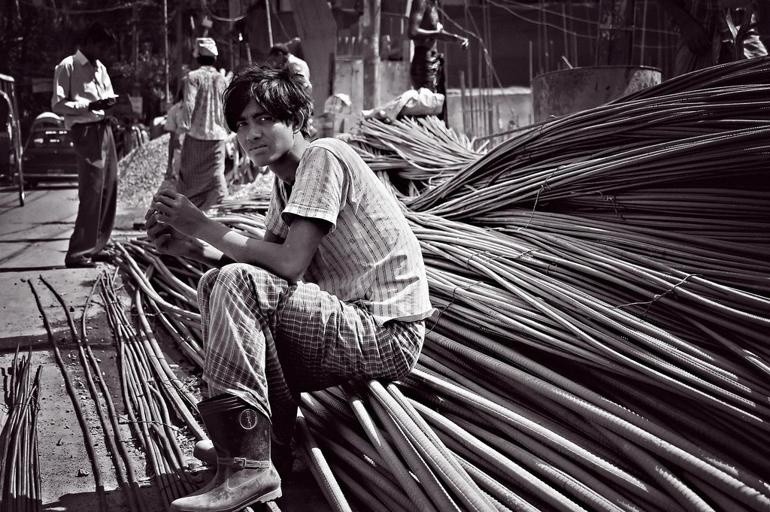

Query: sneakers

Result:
[65,256,97,269]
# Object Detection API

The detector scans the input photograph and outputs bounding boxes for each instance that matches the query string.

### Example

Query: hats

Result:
[192,37,219,56]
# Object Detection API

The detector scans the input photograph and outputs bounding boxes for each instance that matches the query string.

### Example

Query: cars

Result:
[20,111,149,192]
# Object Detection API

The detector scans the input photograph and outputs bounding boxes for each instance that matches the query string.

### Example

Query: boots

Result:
[171,385,283,512]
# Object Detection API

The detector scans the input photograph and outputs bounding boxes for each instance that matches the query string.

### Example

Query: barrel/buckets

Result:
[531,66,662,123]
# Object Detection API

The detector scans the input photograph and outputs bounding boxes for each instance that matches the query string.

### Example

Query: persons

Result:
[656,1,769,81]
[108,116,126,160]
[142,66,439,511]
[50,23,123,267]
[120,113,144,159]
[408,1,470,130]
[268,42,312,95]
[169,36,232,212]
[162,75,188,167]
